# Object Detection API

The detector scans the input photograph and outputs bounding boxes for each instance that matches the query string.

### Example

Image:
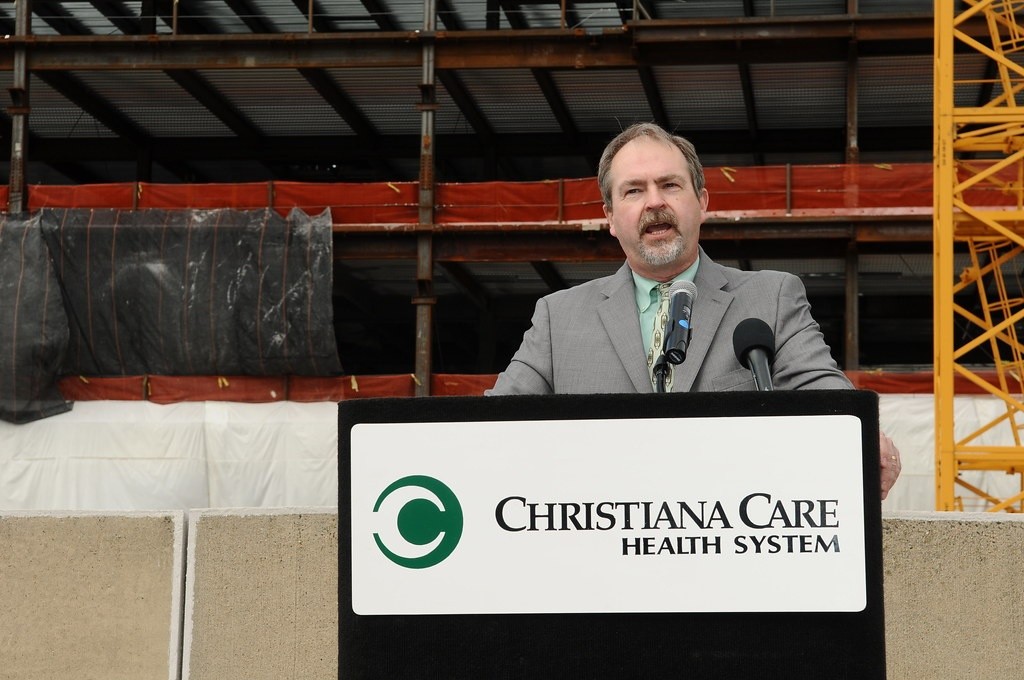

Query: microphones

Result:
[664,280,699,364]
[732,318,777,390]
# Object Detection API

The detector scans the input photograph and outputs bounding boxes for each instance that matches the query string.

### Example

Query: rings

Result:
[891,456,897,460]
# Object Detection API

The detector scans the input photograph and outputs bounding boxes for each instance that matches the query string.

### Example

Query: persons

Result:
[493,124,901,500]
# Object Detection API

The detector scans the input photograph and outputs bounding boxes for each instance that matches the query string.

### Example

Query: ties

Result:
[645,281,674,393]
